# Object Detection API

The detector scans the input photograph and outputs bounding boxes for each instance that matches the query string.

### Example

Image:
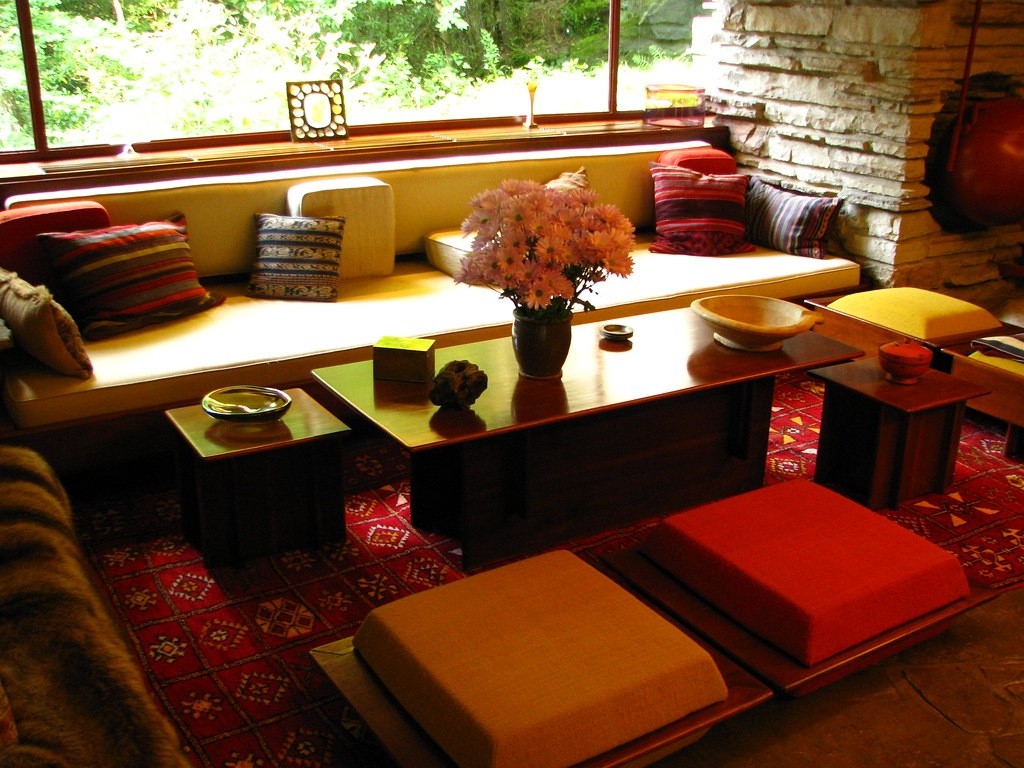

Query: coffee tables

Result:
[165,387,351,569]
[808,357,993,511]
[308,309,866,570]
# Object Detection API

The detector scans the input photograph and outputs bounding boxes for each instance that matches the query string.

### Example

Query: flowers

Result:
[455,178,636,319]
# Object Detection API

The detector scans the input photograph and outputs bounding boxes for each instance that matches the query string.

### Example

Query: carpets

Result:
[79,365,1024,767]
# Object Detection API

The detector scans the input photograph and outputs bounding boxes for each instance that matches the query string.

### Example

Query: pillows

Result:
[647,164,847,256]
[0,200,344,381]
[544,165,590,193]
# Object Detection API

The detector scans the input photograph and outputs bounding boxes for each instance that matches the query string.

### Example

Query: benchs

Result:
[804,286,1024,457]
[308,477,1000,768]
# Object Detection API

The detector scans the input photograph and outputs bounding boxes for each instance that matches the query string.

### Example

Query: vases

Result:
[511,307,573,378]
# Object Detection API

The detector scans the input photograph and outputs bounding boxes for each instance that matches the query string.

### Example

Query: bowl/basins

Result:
[690,293,824,352]
[878,336,934,385]
[203,383,293,423]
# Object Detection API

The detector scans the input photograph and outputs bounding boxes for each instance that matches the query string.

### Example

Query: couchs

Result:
[0,143,859,477]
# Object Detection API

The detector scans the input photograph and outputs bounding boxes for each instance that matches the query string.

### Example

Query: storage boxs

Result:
[373,336,436,382]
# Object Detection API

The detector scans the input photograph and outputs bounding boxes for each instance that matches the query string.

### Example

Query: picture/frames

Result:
[287,80,349,143]
